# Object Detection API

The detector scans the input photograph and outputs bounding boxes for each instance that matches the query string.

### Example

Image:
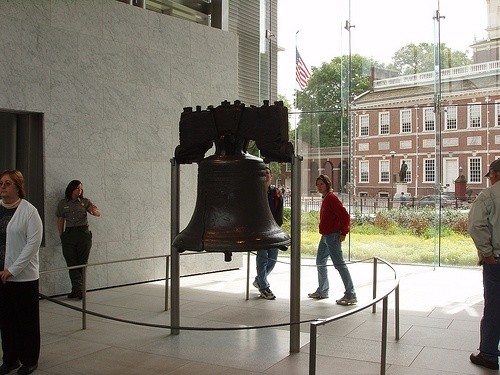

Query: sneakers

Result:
[336,293,357,305]
[259,287,275,299]
[252,278,269,289]
[309,290,328,298]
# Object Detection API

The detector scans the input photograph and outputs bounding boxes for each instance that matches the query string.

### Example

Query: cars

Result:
[406,193,463,209]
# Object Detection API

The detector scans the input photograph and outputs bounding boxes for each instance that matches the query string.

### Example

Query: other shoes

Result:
[68,292,81,299]
[17,364,38,374]
[0,360,20,374]
[470,352,499,370]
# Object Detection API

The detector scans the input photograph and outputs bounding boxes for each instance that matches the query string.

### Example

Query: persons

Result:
[56,180,100,298]
[400,160,409,180]
[308,174,358,305]
[253,167,284,299]
[468,158,500,370]
[0,170,44,375]
[275,185,291,195]
[400,192,407,201]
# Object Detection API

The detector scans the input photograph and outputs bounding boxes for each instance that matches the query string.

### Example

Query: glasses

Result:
[0,180,16,186]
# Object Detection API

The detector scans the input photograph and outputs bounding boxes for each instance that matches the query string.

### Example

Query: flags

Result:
[296,48,311,91]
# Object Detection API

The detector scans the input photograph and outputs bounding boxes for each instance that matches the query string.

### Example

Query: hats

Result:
[484,158,500,178]
